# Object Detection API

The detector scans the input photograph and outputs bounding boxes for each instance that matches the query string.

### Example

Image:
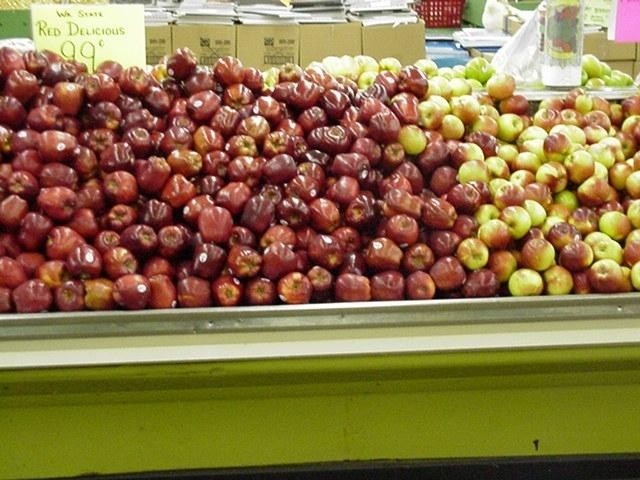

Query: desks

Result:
[0,290,640,476]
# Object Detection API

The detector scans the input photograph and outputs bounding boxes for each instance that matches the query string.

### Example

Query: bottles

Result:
[542,0,584,91]
[535,1,546,75]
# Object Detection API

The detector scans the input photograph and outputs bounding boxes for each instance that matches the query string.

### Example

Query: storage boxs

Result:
[1,11,32,41]
[146,22,173,64]
[633,42,640,78]
[172,24,235,67]
[584,32,636,79]
[299,20,362,69]
[362,16,424,66]
[31,3,147,74]
[236,25,299,70]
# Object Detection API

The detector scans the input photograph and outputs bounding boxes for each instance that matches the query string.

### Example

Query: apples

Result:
[0,45,639,314]
